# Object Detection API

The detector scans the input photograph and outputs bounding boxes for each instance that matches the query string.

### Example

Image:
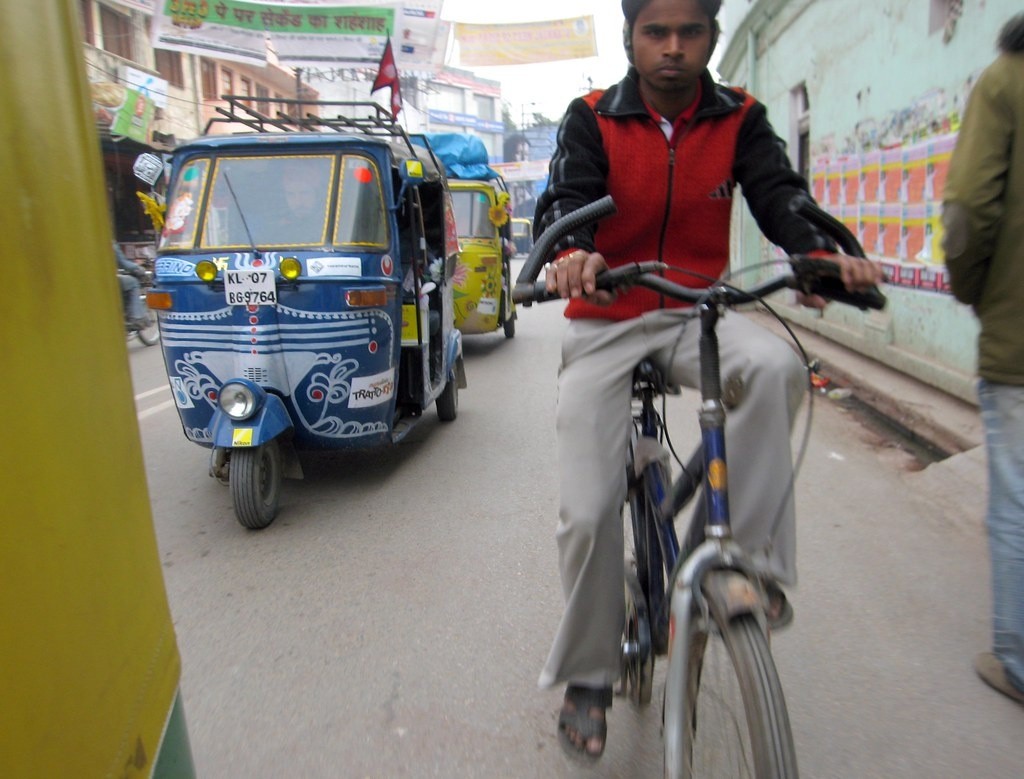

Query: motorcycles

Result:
[146,93,468,533]
[446,171,517,340]
[510,216,535,260]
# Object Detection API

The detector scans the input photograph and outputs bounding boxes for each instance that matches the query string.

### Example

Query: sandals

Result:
[760,578,794,629]
[557,684,614,764]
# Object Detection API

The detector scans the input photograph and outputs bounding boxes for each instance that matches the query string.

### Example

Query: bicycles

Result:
[508,194,889,779]
[128,270,160,345]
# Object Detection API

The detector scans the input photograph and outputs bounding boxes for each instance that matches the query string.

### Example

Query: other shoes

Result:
[973,652,1024,702]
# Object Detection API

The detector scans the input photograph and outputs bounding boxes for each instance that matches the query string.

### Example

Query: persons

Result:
[533,0,881,767]
[500,237,517,286]
[257,161,326,245]
[115,240,152,330]
[941,10,1024,707]
